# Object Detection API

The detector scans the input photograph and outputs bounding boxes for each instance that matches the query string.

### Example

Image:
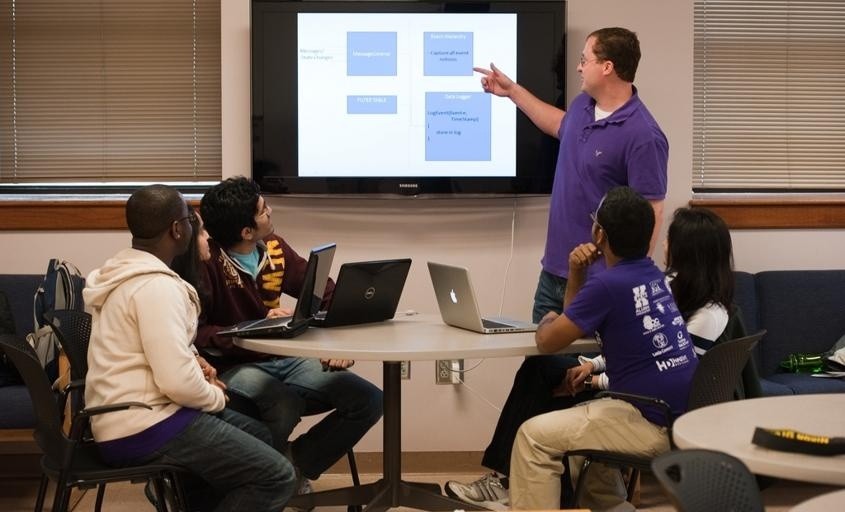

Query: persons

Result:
[144,210,263,512]
[508,187,701,511]
[473,27,671,322]
[199,175,384,511]
[445,207,762,512]
[82,184,298,512]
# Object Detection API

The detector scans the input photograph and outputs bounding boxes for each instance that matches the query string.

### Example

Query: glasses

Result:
[588,210,607,238]
[177,210,197,223]
[580,55,598,66]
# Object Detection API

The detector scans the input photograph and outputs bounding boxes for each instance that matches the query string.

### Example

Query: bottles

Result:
[778,353,823,376]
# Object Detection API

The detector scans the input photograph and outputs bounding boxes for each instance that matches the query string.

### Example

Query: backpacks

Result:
[24,258,82,400]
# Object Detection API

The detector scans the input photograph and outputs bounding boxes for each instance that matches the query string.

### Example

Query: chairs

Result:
[0,274,87,512]
[1,332,188,512]
[648,447,765,512]
[561,326,772,512]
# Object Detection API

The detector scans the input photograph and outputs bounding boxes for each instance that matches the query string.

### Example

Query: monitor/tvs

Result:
[249,0,568,194]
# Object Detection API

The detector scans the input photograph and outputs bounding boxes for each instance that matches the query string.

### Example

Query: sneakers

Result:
[445,472,510,510]
[281,440,315,512]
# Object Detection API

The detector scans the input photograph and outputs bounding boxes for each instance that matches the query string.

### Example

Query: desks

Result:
[229,305,604,512]
[671,391,845,489]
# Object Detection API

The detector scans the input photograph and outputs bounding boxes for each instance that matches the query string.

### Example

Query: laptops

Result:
[309,258,412,328]
[427,261,539,334]
[216,243,336,337]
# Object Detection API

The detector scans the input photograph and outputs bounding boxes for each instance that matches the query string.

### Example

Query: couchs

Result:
[718,268,845,397]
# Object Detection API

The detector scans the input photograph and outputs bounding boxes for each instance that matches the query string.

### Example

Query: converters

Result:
[451,360,460,384]
